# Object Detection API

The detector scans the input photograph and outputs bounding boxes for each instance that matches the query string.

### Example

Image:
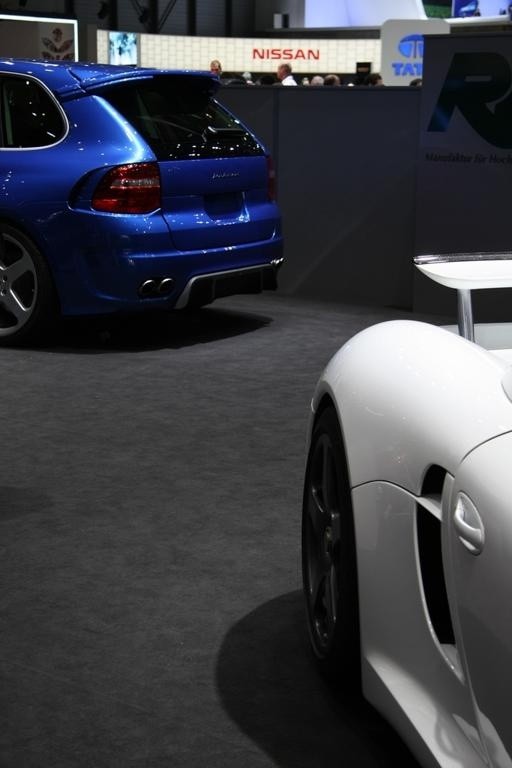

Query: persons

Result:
[210,60,383,87]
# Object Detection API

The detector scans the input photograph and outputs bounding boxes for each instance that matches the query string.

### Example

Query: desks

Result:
[412,251,511,342]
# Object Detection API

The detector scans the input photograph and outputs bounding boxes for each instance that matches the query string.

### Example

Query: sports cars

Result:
[301,250,512,768]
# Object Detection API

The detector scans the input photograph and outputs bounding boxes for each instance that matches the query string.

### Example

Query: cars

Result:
[0,58,286,346]
